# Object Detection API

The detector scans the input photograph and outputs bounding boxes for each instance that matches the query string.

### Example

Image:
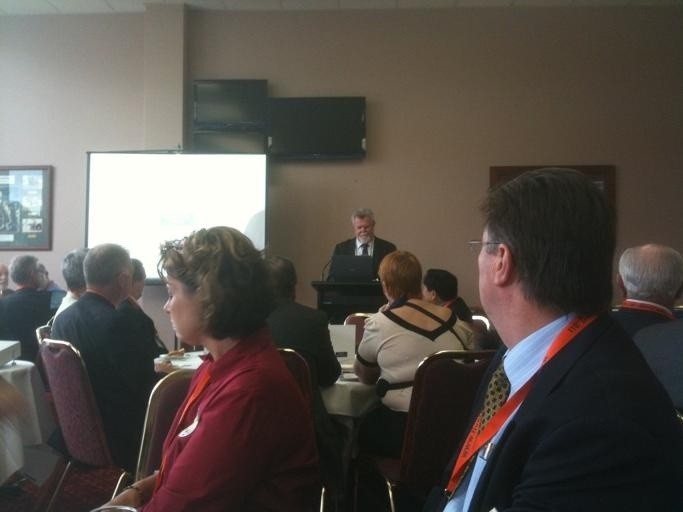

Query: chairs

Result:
[107,371,343,512]
[38,339,121,510]
[372,349,494,503]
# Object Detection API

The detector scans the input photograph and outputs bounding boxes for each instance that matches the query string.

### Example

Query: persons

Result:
[50,243,149,420]
[417,264,465,307]
[421,168,680,512]
[353,250,480,454]
[50,247,86,332]
[121,256,166,357]
[261,254,339,391]
[0,255,63,362]
[0,264,9,295]
[613,242,682,414]
[85,227,320,510]
[327,208,399,283]
[35,264,63,294]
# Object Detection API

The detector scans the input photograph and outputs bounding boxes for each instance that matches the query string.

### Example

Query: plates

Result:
[340,364,359,381]
[158,353,191,359]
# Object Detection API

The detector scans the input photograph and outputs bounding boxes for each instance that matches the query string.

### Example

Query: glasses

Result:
[468,240,501,256]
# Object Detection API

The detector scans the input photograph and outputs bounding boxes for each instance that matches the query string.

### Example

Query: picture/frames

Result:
[0,165,54,253]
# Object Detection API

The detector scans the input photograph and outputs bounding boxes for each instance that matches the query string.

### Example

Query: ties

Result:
[361,244,369,255]
[477,363,510,432]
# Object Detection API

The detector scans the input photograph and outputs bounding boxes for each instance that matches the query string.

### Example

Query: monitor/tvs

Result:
[335,255,371,282]
[192,79,268,133]
[266,97,366,161]
[490,164,616,253]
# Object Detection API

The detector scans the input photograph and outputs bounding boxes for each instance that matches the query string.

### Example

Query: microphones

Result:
[321,255,335,282]
